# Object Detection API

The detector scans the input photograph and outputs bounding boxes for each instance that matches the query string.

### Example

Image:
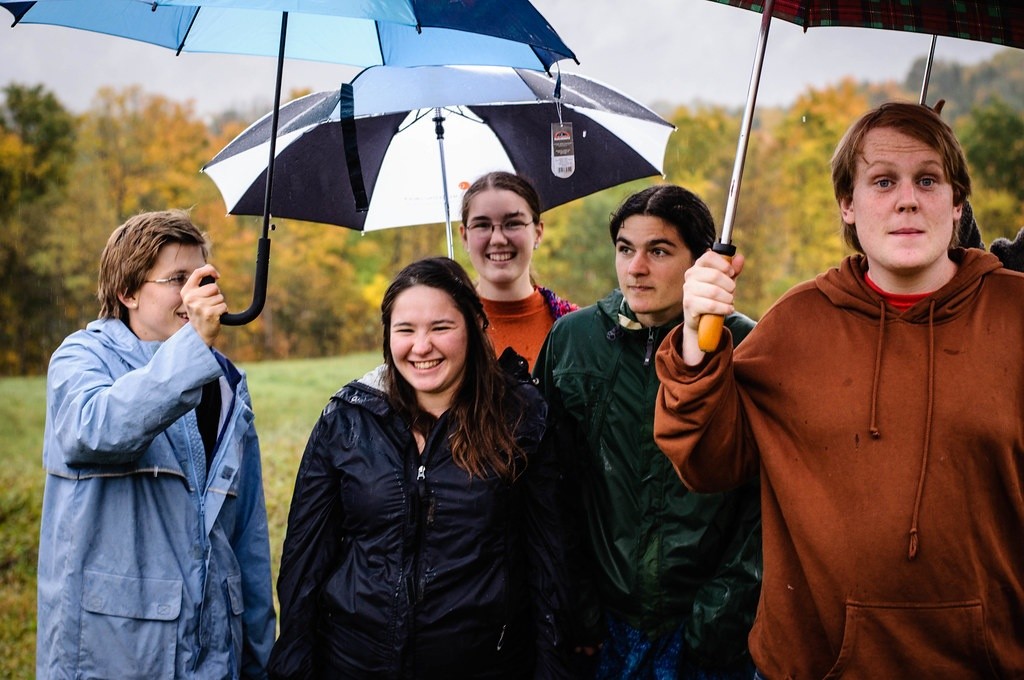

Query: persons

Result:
[916,98,1024,272]
[449,170,582,382]
[531,185,762,679]
[652,103,1023,680]
[30,206,277,680]
[265,255,551,680]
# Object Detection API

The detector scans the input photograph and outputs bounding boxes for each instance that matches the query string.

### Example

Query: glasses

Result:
[465,220,535,235]
[144,274,189,285]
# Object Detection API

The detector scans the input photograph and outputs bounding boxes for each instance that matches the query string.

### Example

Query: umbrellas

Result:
[688,0,1024,357]
[0,1,583,326]
[199,62,678,266]
[917,35,939,108]
